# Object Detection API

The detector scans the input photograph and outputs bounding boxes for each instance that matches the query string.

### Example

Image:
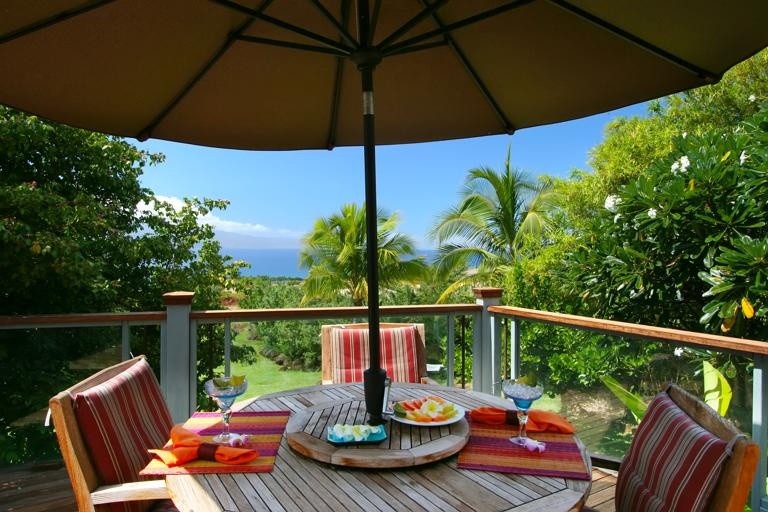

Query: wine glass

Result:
[205,376,248,443]
[502,375,543,445]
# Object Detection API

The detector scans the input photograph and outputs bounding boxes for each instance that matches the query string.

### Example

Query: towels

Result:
[469,405,577,435]
[146,423,259,468]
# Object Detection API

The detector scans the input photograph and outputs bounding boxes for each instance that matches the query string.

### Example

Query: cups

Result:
[382,376,391,413]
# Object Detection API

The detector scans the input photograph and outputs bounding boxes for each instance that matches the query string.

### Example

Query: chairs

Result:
[581,385,761,512]
[48,354,179,512]
[321,322,439,386]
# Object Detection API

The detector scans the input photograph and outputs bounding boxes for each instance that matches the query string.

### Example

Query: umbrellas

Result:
[0,0,767,426]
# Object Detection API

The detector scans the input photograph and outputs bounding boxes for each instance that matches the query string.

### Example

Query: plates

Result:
[390,398,465,425]
